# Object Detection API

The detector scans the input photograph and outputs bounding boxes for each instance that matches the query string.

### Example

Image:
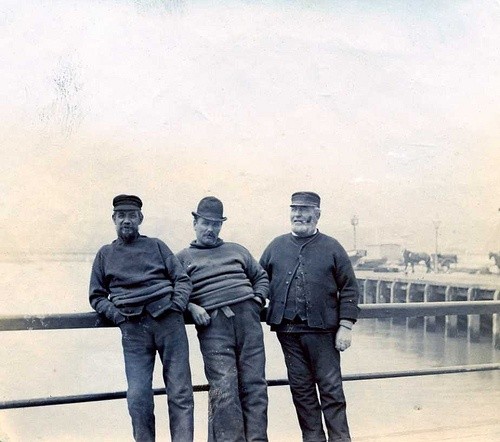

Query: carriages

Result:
[402,247,458,275]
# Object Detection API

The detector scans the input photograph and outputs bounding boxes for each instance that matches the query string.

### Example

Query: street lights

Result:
[350,215,359,249]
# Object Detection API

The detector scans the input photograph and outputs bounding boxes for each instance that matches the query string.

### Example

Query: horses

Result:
[402,248,432,274]
[488,253,500,269]
[430,253,458,270]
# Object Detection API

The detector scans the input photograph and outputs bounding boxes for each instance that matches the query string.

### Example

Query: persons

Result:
[260,192,361,442]
[89,195,194,442]
[174,196,270,442]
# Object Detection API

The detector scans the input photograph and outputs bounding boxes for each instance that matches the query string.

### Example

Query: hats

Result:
[191,196,227,222]
[290,191,321,208]
[113,194,142,211]
